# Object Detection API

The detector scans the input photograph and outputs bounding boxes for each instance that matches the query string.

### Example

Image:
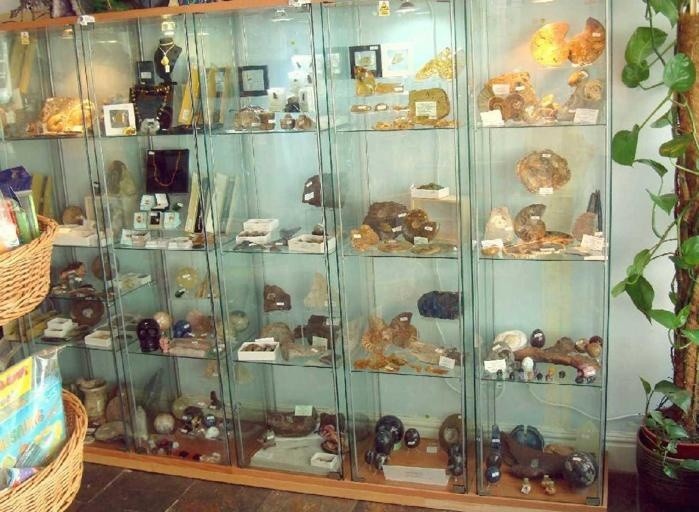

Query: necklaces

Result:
[126,83,174,135]
[147,149,186,190]
[155,39,179,72]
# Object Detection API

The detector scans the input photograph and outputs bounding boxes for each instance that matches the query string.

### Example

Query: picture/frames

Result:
[237,65,268,96]
[348,46,381,78]
[104,102,136,136]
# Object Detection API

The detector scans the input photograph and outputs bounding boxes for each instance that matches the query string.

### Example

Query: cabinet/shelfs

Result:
[0,0,615,512]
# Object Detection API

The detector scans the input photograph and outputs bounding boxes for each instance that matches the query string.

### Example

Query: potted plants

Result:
[612,0,699,512]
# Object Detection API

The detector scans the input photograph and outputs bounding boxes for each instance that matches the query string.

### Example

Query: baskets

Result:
[0,384,87,512]
[1,214,60,326]
